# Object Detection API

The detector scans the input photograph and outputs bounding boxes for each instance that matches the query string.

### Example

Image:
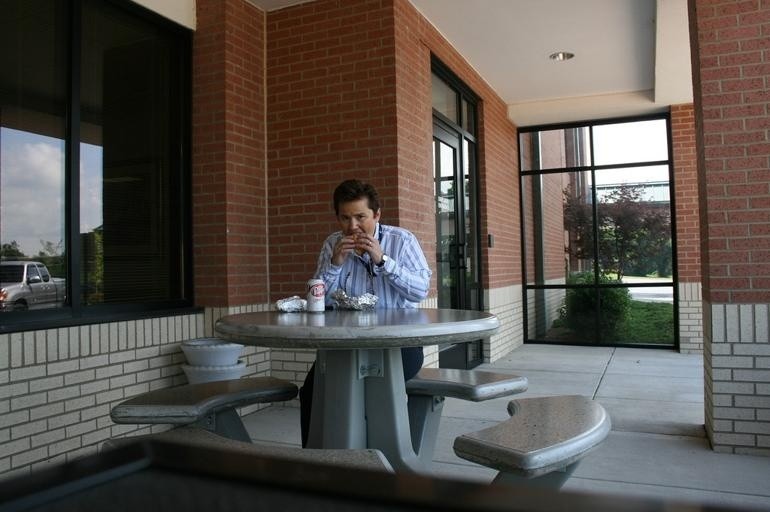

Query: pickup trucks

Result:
[0,262,67,313]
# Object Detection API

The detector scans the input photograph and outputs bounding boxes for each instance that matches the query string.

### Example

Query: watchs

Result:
[377,254,389,267]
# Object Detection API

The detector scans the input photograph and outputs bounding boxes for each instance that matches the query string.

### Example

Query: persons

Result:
[300,179,433,451]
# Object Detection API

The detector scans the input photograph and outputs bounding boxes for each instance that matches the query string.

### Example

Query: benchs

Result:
[401,367,529,467]
[454,394,613,494]
[109,376,299,443]
[143,422,401,502]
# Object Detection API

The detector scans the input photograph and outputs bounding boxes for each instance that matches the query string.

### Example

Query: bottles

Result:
[307,279,328,314]
